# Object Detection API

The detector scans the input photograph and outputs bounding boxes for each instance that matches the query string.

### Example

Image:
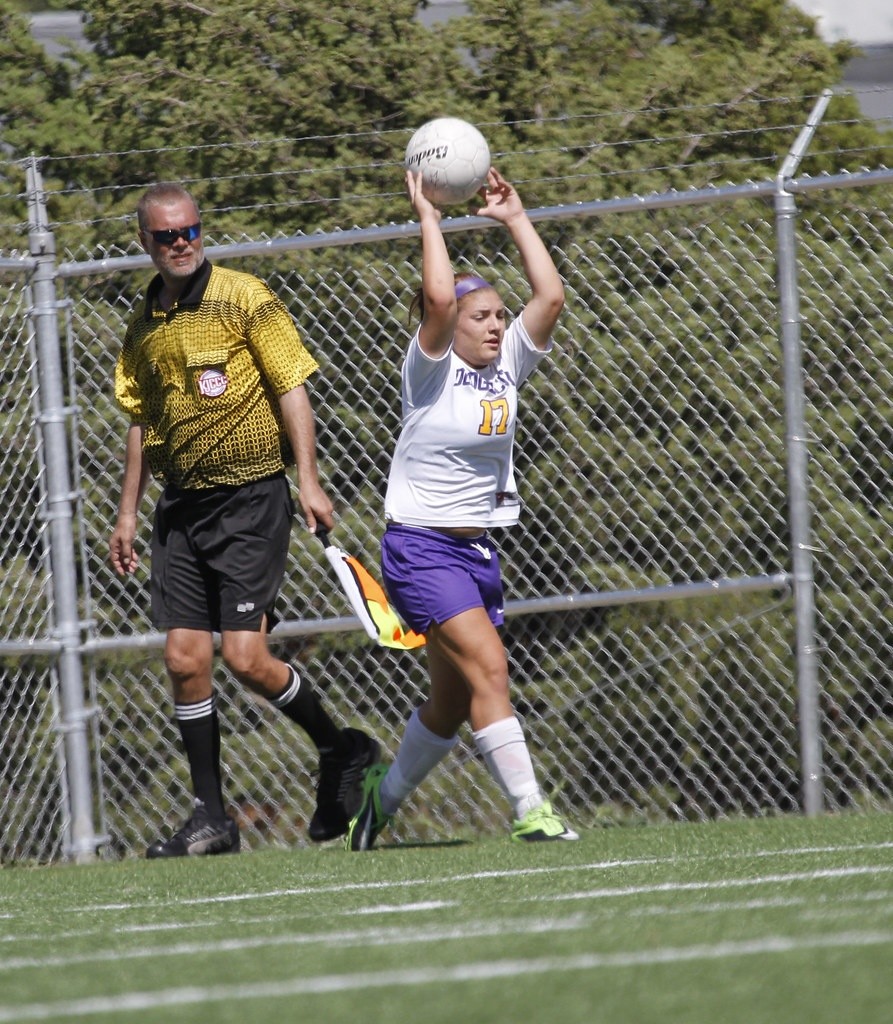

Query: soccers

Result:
[405,117,491,206]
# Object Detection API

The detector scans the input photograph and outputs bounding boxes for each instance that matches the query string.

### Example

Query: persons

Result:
[344,168,577,852]
[109,183,378,861]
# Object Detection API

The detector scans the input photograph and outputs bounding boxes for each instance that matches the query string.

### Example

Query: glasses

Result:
[143,221,201,245]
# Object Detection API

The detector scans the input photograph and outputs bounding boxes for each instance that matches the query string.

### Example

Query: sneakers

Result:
[309,728,381,841]
[344,762,393,852]
[508,800,579,846]
[147,797,241,859]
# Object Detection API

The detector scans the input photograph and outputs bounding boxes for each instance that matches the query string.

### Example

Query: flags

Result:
[324,544,427,649]
